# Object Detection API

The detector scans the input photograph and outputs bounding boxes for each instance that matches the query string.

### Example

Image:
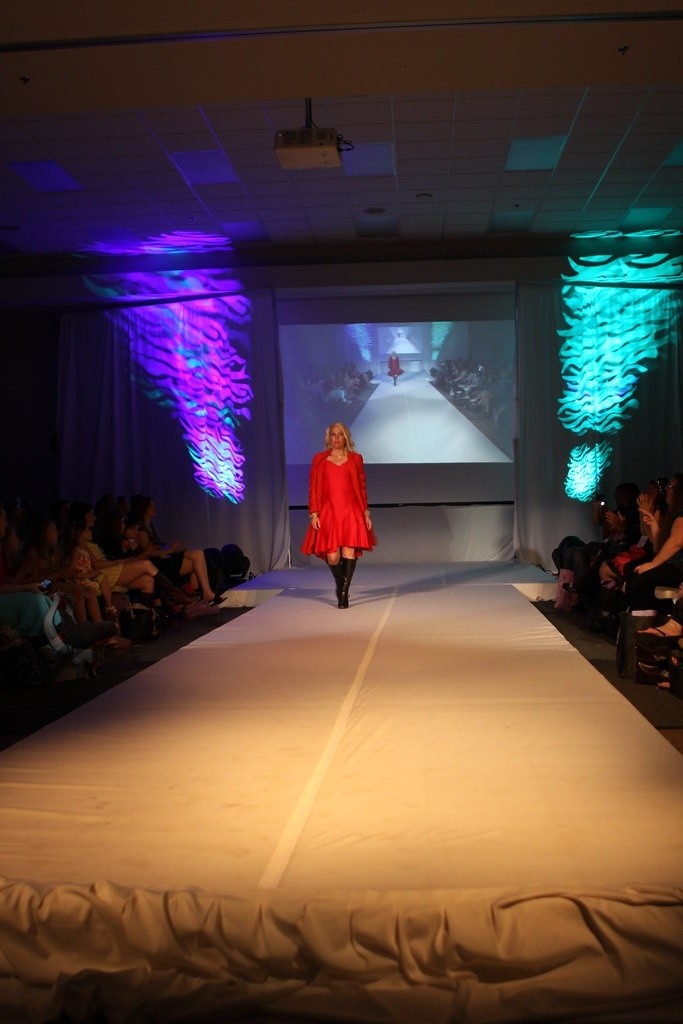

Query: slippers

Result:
[209,595,230,607]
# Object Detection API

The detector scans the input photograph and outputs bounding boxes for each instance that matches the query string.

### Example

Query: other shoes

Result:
[637,627,683,641]
[636,641,672,657]
[601,611,612,621]
[638,661,665,676]
[562,581,579,593]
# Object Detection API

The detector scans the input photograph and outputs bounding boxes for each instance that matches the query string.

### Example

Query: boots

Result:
[326,557,343,600]
[154,571,202,613]
[338,555,357,609]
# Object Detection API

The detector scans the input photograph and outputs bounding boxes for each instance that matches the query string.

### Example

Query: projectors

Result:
[273,128,343,170]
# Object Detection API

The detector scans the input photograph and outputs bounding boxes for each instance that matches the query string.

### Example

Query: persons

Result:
[285,361,367,416]
[387,351,405,386]
[301,422,378,608]
[562,473,683,689]
[443,352,513,418]
[0,493,228,665]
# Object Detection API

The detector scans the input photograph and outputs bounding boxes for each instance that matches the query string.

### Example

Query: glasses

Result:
[665,482,679,492]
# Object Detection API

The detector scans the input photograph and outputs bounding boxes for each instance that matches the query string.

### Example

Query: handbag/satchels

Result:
[119,607,158,640]
[0,640,56,690]
[615,610,659,680]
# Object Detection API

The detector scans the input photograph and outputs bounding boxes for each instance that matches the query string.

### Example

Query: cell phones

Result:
[40,578,53,589]
